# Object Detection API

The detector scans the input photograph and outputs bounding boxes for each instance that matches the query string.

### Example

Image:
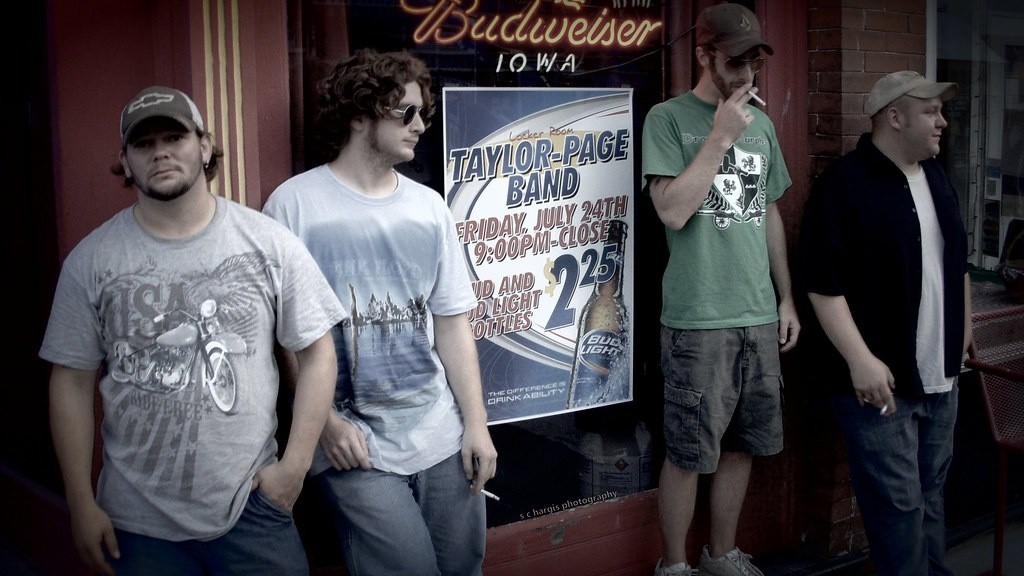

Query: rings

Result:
[864,398,871,402]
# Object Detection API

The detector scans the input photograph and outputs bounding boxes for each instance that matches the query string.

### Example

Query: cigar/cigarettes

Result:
[470,485,500,501]
[880,404,887,414]
[747,90,766,106]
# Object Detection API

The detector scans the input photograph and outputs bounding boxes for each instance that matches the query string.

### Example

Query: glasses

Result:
[384,105,426,126]
[707,51,765,74]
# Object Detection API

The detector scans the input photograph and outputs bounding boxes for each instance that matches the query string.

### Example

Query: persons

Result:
[259,50,498,576]
[38,85,347,576]
[800,70,972,576]
[642,4,801,576]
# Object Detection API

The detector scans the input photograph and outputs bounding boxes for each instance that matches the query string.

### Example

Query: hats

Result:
[696,3,775,58]
[121,86,205,151]
[865,72,959,117]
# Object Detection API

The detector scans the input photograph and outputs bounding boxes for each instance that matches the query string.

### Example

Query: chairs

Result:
[964,304,1024,576]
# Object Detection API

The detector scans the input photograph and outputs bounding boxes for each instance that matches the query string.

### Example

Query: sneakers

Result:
[656,558,699,576]
[701,545,764,576]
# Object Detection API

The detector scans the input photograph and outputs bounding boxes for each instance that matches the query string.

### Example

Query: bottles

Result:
[568,219,628,411]
